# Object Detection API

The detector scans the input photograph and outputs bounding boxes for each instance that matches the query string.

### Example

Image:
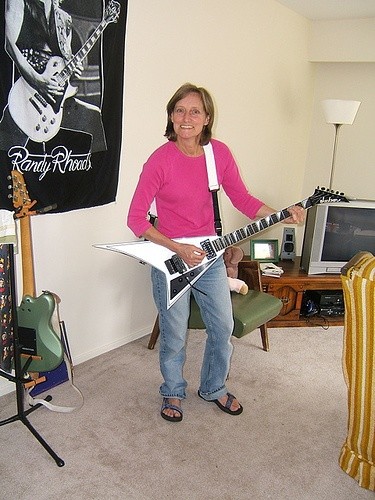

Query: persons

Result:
[0,0,108,156]
[126,82,305,422]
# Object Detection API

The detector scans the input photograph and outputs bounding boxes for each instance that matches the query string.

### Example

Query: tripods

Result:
[0,244,65,467]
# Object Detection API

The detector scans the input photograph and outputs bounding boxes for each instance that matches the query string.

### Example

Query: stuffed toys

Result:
[223,247,249,296]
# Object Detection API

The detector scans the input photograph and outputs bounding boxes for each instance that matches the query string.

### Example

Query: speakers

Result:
[281,227,296,259]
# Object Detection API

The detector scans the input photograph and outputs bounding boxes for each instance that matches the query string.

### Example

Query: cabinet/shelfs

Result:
[238,256,346,327]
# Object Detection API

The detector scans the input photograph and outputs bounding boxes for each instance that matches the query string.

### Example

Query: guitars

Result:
[8,0,121,143]
[7,169,64,375]
[92,186,350,310]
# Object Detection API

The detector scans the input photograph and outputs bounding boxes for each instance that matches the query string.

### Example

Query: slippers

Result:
[197,389,243,415]
[160,397,183,422]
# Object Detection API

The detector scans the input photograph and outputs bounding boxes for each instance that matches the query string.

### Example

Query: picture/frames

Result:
[249,238,279,264]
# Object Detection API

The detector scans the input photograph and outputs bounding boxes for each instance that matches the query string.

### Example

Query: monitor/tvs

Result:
[299,202,375,276]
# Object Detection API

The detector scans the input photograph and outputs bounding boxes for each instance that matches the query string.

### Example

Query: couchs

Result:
[340,252,375,490]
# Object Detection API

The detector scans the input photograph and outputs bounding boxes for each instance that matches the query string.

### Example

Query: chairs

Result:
[147,218,283,351]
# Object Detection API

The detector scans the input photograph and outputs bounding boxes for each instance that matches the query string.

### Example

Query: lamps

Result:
[320,99,362,189]
